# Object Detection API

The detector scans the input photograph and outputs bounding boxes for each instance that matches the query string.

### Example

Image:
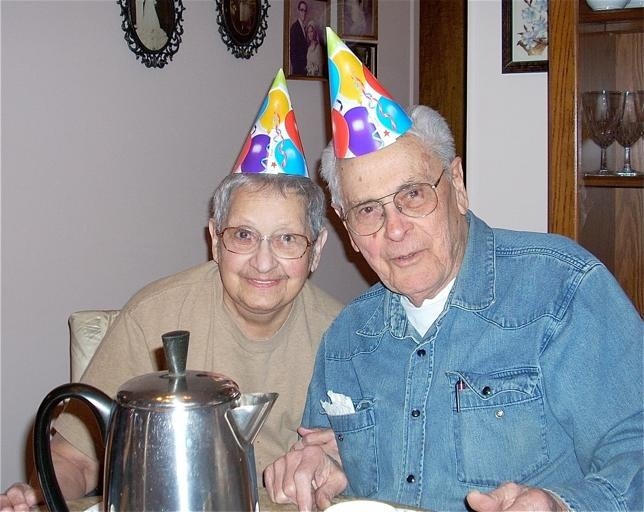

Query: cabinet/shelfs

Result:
[547,1,644,321]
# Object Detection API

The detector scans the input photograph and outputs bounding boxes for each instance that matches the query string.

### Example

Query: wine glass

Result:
[580,90,644,176]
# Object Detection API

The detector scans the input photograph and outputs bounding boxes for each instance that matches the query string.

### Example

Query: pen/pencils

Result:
[455,378,465,412]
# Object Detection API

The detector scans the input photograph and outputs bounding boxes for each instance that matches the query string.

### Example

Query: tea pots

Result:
[33,331,279,512]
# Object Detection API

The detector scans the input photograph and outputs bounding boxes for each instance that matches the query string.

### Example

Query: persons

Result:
[264,25,643,512]
[0,68,342,510]
[290,0,324,79]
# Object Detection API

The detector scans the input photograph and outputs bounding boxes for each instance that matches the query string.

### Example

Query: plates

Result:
[79,502,102,511]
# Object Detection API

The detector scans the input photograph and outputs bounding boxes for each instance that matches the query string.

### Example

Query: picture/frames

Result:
[502,1,549,72]
[283,0,379,81]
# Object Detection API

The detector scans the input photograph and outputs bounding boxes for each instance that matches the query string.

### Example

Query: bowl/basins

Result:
[584,0,630,11]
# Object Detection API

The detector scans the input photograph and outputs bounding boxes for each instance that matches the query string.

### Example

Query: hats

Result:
[230,68,310,178]
[326,26,415,159]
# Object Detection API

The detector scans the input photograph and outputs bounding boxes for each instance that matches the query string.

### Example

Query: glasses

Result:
[340,168,446,235]
[216,225,318,260]
